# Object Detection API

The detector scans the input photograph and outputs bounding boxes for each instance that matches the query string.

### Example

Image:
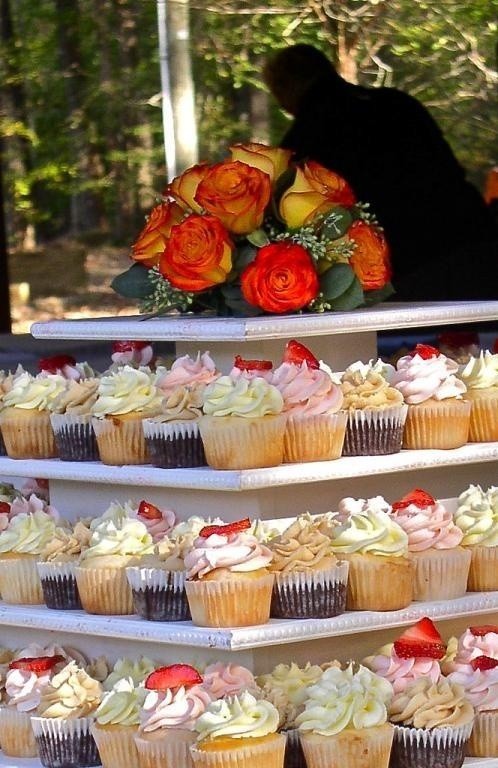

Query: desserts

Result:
[0,341,498,469]
[0,482,498,627]
[0,617,498,768]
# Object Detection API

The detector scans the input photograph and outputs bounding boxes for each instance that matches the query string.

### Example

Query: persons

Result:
[261,45,497,302]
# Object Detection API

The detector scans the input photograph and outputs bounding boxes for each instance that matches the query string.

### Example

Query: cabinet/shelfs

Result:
[0,302,497,767]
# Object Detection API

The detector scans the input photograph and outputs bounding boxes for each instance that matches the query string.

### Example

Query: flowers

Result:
[110,140,396,320]
